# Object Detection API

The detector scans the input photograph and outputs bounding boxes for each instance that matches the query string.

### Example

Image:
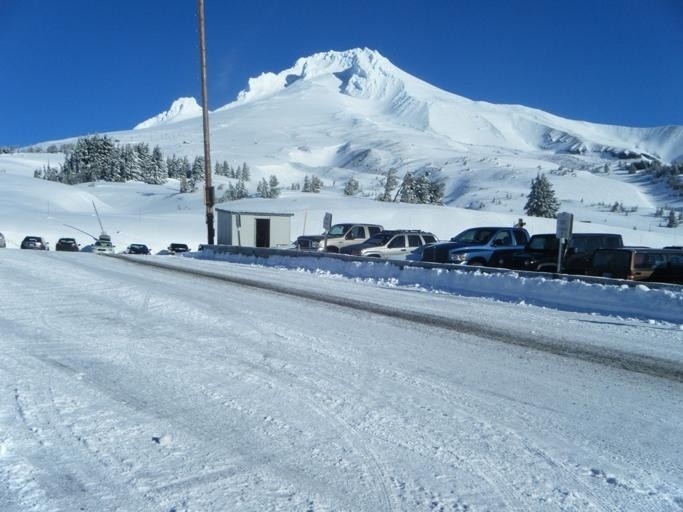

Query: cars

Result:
[90,240,115,256]
[127,244,151,255]
[0,232,6,247]
[56,238,80,251]
[168,243,190,255]
[22,236,49,250]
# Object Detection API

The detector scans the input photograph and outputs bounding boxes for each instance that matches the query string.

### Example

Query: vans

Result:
[584,246,683,284]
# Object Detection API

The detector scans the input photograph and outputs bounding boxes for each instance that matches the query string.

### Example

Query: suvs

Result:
[297,223,384,254]
[340,230,440,262]
[423,227,531,268]
[498,234,624,276]
